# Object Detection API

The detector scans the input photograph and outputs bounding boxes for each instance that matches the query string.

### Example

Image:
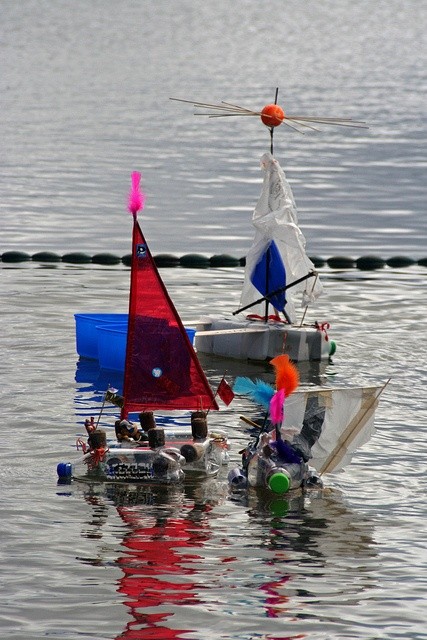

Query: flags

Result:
[282,386,381,478]
[238,151,326,326]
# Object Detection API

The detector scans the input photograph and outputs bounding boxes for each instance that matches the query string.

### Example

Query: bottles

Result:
[256,449,305,494]
[320,333,337,360]
[139,429,230,463]
[55,447,185,485]
[101,438,226,474]
